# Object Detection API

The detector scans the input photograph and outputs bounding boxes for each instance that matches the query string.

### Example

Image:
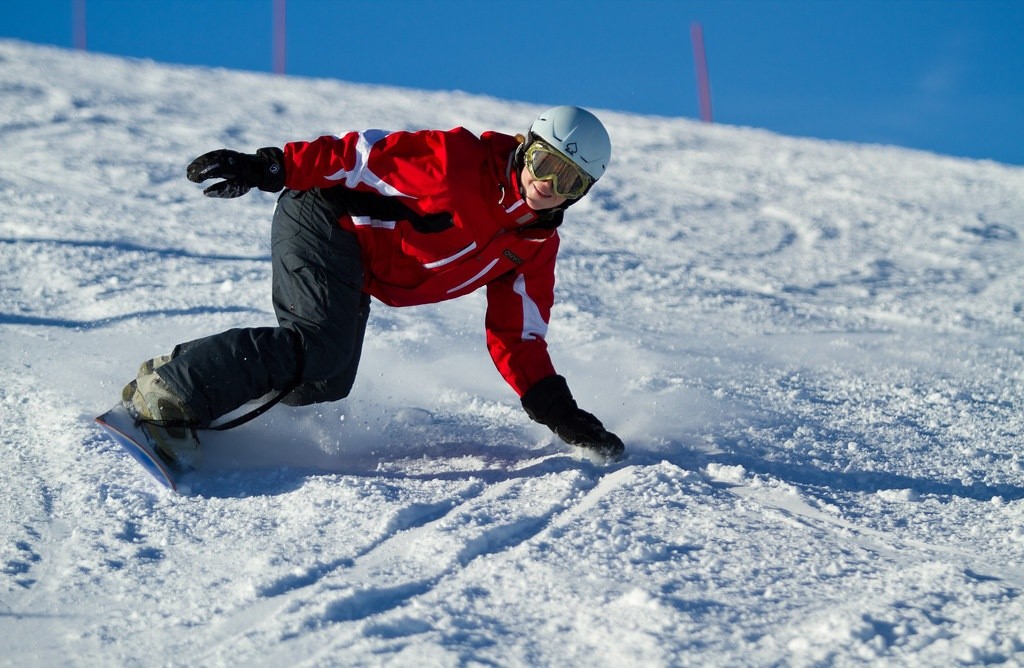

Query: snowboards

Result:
[94,385,186,492]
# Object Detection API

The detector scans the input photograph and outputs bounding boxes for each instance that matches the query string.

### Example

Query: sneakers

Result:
[137,354,171,377]
[122,374,203,472]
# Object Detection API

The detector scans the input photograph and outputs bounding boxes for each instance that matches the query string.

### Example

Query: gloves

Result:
[187,146,284,200]
[520,375,625,459]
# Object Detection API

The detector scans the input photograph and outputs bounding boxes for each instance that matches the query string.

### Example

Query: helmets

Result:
[530,105,611,180]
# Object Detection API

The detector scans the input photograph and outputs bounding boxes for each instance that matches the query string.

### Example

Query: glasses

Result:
[522,141,587,199]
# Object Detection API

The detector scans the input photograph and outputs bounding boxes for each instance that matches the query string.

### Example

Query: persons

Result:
[121,104,629,468]
